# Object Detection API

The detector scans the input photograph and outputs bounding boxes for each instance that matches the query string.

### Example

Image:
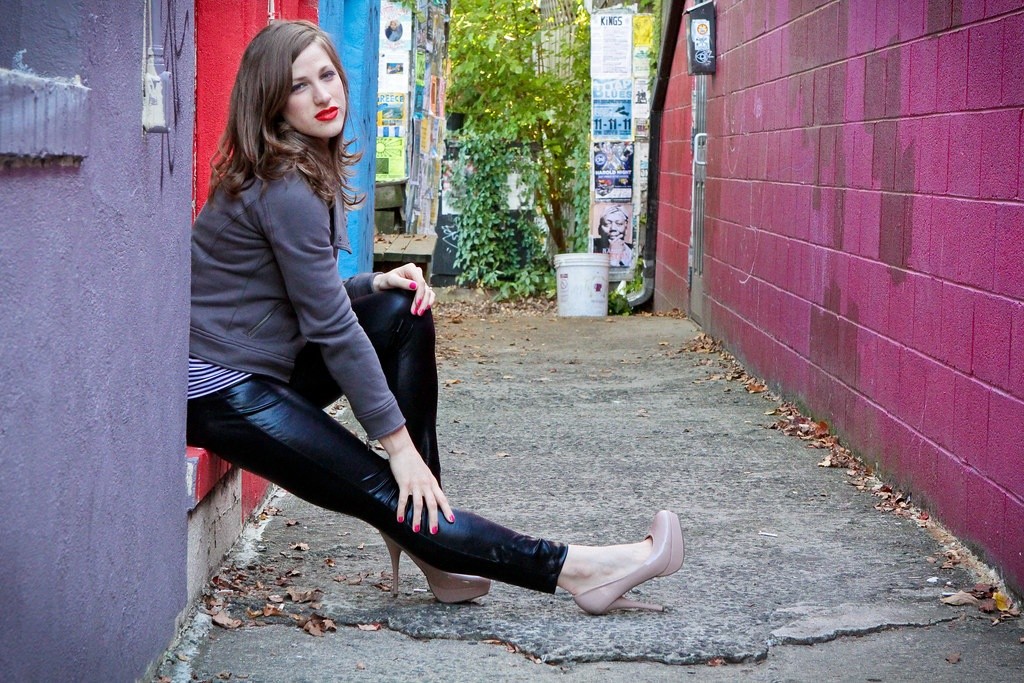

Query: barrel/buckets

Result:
[553,252,609,318]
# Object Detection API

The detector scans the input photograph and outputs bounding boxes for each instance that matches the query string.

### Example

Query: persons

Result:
[187,21,684,616]
[592,205,632,268]
[385,21,400,42]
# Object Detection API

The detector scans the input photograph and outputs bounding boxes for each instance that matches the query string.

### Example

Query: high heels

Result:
[572,509,685,615]
[379,528,491,604]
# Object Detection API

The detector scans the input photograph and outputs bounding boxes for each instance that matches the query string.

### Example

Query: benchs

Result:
[372,176,439,271]
